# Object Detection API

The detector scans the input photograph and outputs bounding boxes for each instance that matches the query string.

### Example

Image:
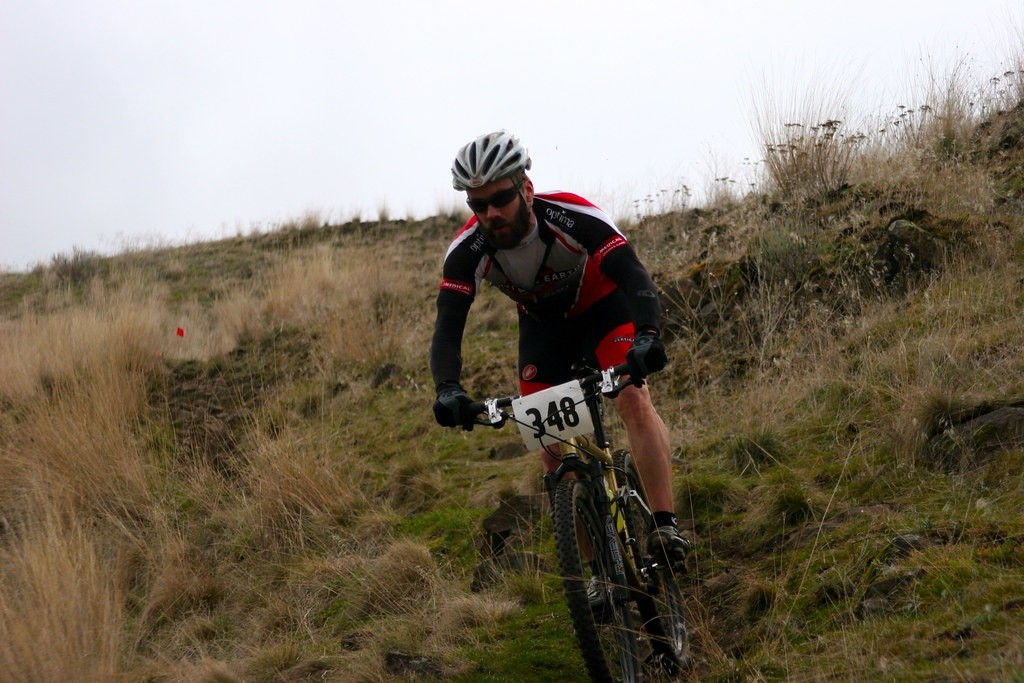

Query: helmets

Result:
[452,132,532,191]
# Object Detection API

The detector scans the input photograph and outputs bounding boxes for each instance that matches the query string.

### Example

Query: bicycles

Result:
[461,361,692,683]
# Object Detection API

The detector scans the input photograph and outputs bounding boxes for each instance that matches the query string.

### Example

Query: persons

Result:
[431,128,693,616]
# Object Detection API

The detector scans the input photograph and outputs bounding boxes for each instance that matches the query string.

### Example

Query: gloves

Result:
[433,386,478,432]
[626,332,667,388]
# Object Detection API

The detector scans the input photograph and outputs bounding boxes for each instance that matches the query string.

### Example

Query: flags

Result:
[177,327,184,337]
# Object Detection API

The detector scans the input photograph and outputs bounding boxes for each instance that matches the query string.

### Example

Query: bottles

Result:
[604,479,625,534]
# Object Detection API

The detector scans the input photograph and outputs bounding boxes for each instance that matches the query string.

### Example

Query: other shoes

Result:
[568,575,611,624]
[648,527,690,585]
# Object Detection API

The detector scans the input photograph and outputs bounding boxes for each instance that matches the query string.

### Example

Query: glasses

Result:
[467,180,525,213]
